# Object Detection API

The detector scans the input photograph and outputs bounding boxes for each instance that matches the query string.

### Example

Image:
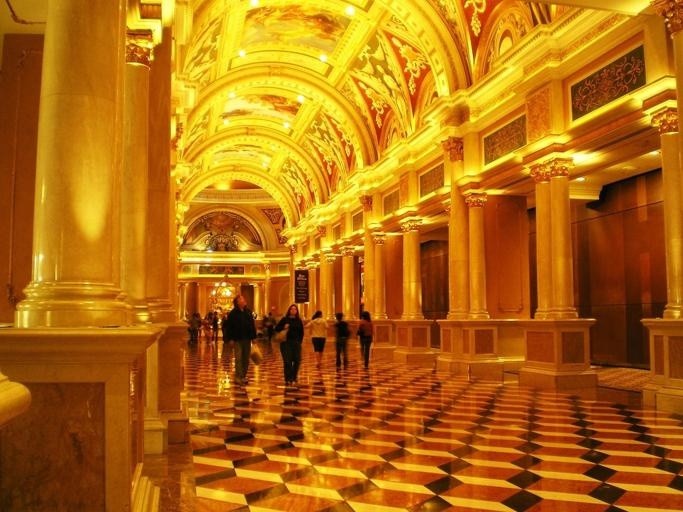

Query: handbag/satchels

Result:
[272,329,288,343]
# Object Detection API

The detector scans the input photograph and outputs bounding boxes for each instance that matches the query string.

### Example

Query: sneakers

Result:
[242,378,249,383]
[235,377,242,385]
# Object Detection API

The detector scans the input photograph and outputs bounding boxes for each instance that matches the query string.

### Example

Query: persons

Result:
[304,309,328,366]
[332,367,349,421]
[223,381,255,469]
[309,367,327,418]
[331,312,351,368]
[221,295,257,386]
[356,310,374,368]
[273,304,304,383]
[188,309,280,346]
[353,367,374,416]
[279,381,304,446]
